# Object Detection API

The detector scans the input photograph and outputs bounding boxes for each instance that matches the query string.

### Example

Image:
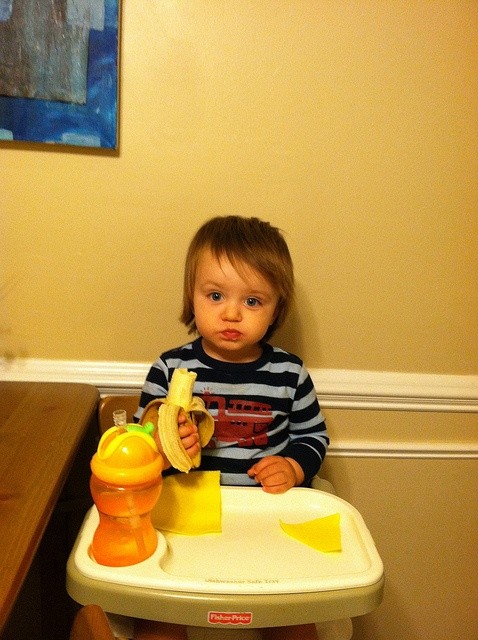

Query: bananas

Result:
[138,367,216,474]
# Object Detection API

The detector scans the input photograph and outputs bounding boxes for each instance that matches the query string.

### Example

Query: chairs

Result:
[67,394,386,638]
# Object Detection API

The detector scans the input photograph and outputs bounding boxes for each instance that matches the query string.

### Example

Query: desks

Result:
[0,383,102,631]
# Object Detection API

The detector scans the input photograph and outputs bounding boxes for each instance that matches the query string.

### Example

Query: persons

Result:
[133,216,329,494]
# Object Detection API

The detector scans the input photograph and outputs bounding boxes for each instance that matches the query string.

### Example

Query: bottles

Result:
[90,410,163,568]
